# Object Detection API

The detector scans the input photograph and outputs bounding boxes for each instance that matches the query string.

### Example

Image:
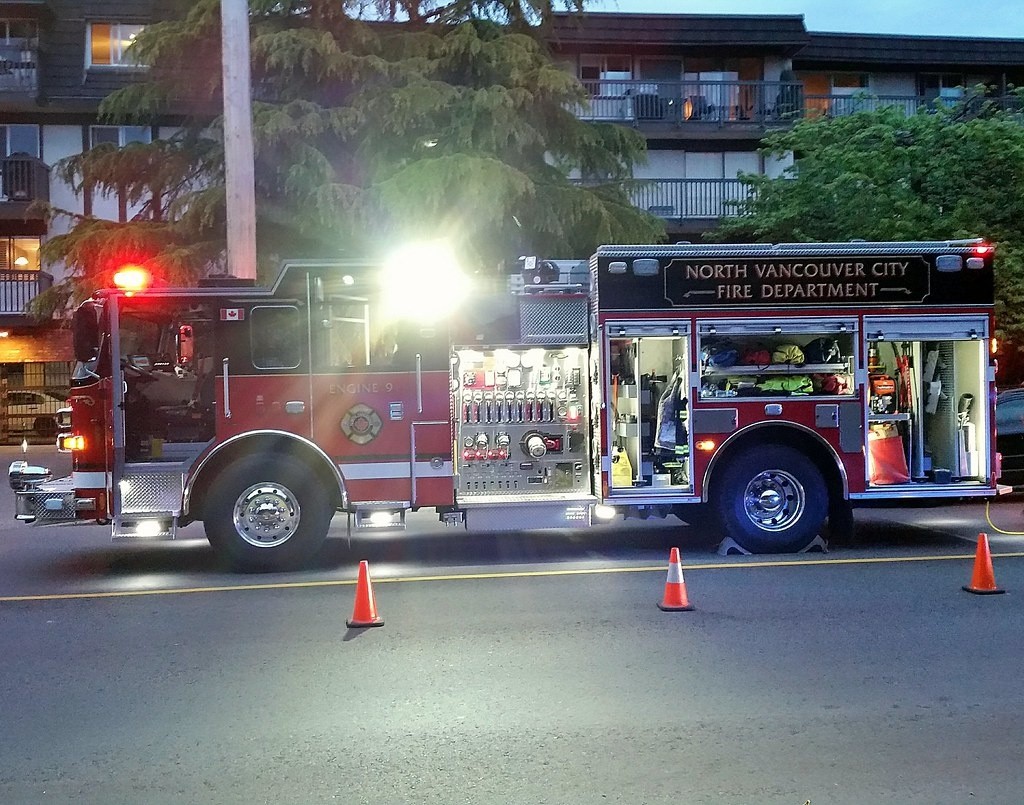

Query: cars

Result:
[7,390,72,437]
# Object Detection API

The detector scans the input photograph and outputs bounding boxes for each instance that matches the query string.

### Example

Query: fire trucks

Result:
[8,239,1013,571]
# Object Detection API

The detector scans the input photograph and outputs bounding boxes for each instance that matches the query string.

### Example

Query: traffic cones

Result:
[346,561,385,627]
[961,533,1006,594]
[656,548,696,611]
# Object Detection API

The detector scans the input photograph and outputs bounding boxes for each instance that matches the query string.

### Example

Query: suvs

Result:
[996,388,1024,490]
